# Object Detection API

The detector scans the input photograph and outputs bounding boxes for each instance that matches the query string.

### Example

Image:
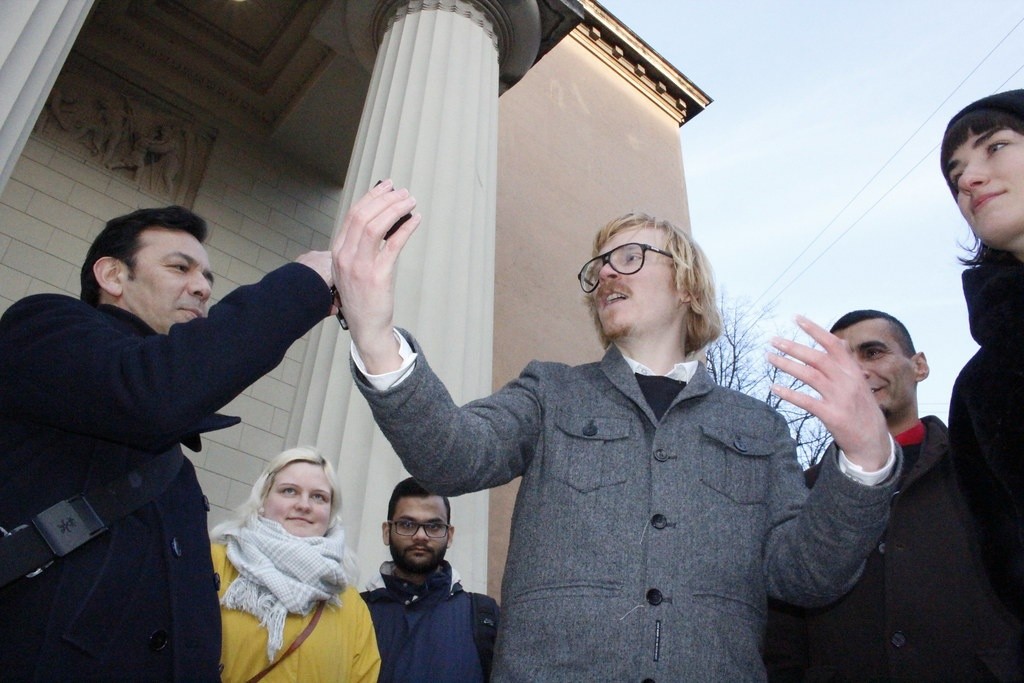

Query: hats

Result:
[945,89,1024,134]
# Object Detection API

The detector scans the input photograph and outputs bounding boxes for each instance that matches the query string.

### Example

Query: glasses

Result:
[578,243,674,293]
[388,520,449,538]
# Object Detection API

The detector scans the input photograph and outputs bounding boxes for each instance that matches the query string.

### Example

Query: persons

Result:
[0,208,331,683]
[210,448,384,683]
[763,88,1024,683]
[359,477,500,683]
[330,175,905,683]
